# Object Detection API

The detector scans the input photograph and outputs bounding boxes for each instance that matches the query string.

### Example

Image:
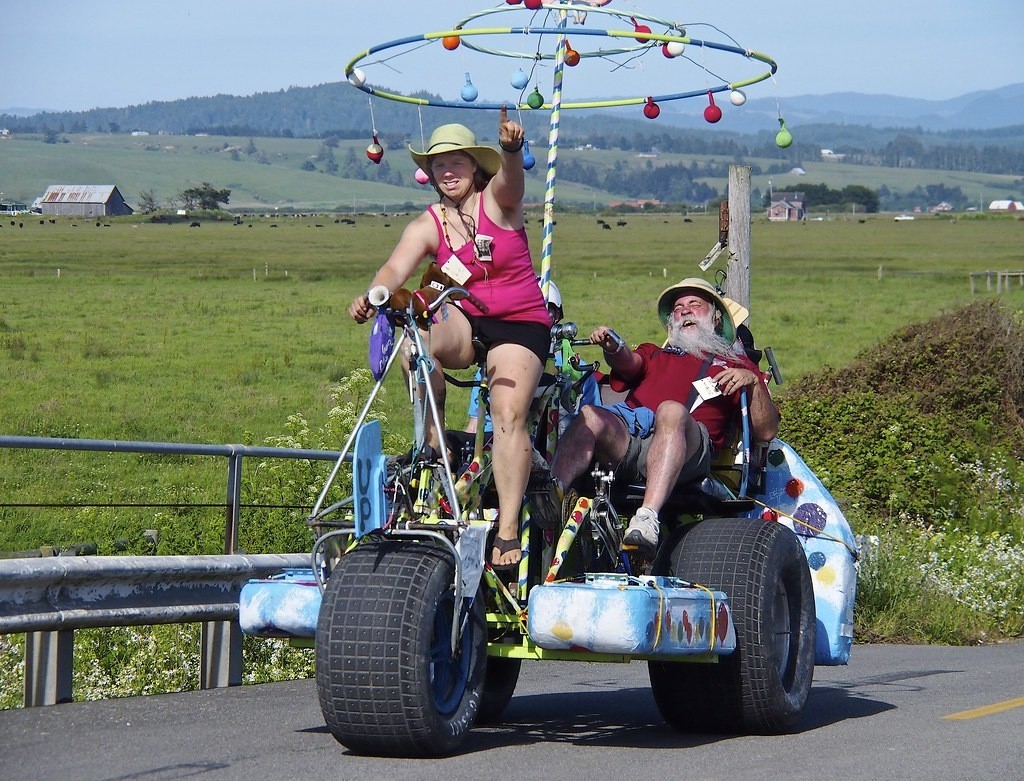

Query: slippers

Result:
[488,533,521,570]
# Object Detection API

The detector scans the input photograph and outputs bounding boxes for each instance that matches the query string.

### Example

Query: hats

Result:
[658,278,736,344]
[407,124,501,176]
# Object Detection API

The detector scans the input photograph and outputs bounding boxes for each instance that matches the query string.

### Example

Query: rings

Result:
[730,380,736,385]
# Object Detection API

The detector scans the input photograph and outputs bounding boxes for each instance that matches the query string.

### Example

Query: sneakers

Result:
[623,507,659,562]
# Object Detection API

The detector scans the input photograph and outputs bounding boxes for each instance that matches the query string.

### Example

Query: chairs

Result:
[540,371,771,578]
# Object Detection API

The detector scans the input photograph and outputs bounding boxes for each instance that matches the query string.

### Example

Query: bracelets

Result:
[603,348,620,355]
[498,137,525,153]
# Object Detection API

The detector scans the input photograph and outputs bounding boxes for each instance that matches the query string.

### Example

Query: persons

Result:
[347,105,556,570]
[529,276,785,551]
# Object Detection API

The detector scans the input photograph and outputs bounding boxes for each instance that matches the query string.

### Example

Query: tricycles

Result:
[239,284,859,753]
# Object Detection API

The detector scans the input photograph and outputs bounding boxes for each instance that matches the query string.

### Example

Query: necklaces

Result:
[439,198,478,266]
